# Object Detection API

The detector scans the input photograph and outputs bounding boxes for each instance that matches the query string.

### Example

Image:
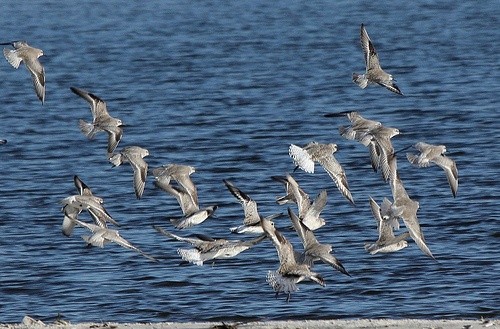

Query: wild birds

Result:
[59,112,436,303]
[352,23,405,97]
[406,142,459,199]
[69,86,127,159]
[1,40,47,106]
